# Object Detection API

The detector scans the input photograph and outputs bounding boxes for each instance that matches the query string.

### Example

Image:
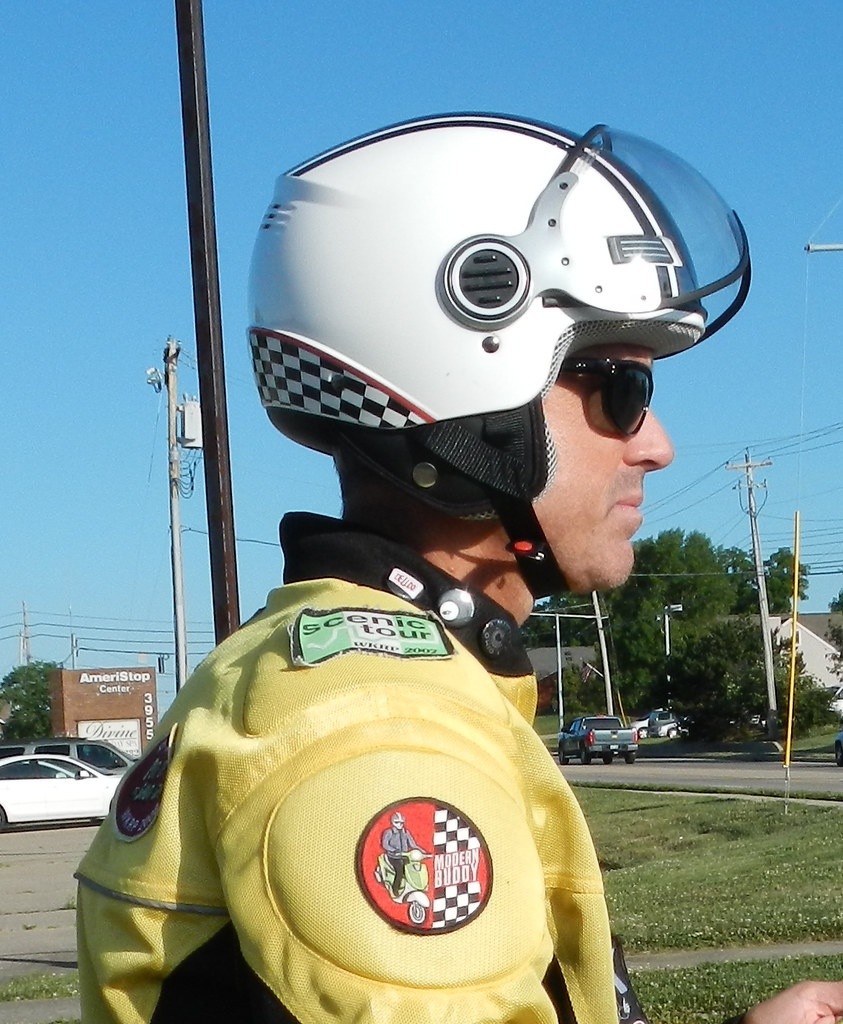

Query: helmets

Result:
[247,109,706,438]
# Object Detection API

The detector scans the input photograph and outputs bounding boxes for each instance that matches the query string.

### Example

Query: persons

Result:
[73,109,842,1024]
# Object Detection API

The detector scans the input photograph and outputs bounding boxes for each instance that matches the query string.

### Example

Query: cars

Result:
[631,707,673,739]
[658,717,687,738]
[0,754,124,831]
[826,684,843,712]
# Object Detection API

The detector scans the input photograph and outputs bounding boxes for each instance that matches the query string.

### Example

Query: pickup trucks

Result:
[558,716,639,764]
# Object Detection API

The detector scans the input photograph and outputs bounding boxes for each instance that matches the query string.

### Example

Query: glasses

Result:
[561,356,654,436]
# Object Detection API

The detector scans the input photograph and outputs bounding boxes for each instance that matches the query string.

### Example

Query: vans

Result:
[0,740,138,775]
